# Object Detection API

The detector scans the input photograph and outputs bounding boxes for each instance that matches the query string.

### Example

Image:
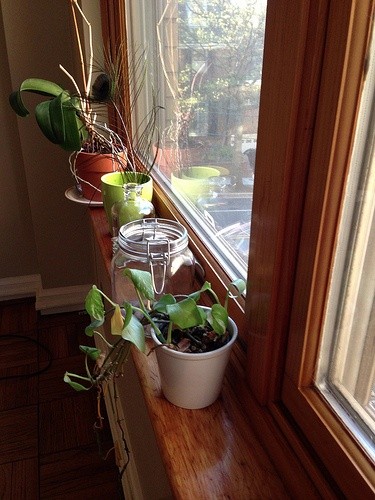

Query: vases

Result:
[110,218,196,306]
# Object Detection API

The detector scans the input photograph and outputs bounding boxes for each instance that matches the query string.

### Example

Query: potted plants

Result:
[8,0,165,235]
[64,267,245,489]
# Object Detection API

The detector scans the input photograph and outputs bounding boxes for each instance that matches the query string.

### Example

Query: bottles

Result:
[110,182,156,235]
[197,176,236,229]
[109,218,195,327]
